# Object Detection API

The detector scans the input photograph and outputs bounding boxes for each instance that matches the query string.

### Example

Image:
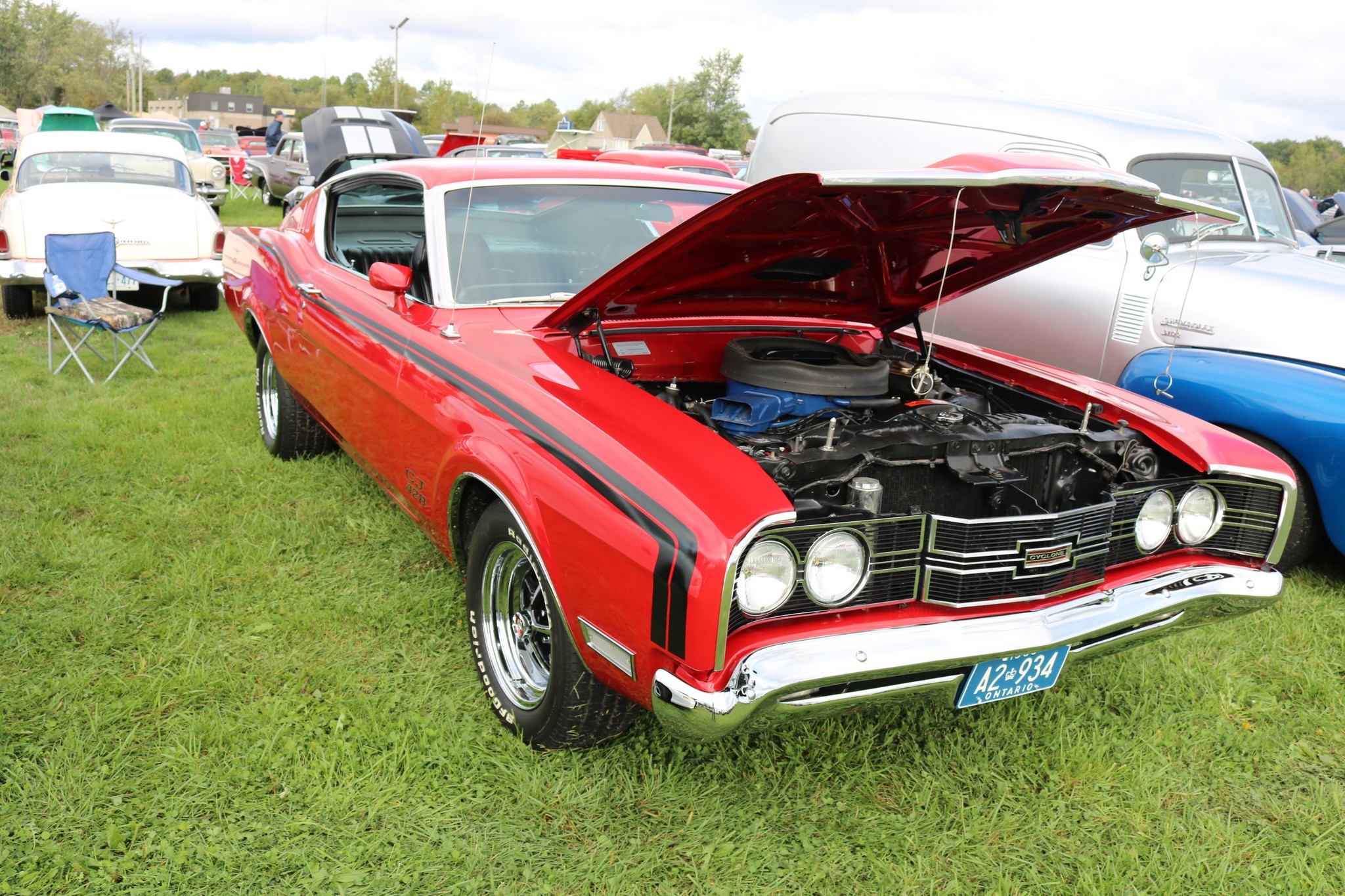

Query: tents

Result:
[92,102,135,120]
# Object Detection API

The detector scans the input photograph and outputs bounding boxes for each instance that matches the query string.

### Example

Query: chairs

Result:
[43,231,183,384]
[228,156,262,200]
[409,231,492,305]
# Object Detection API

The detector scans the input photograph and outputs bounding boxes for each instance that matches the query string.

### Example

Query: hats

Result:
[200,121,206,126]
[275,110,288,116]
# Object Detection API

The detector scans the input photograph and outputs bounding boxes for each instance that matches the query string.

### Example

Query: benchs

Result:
[346,245,602,276]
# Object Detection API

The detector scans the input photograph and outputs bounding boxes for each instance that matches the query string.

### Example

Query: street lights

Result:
[389,17,410,109]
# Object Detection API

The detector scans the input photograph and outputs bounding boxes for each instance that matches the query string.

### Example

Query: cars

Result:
[740,98,1345,573]
[217,156,1298,754]
[0,105,746,321]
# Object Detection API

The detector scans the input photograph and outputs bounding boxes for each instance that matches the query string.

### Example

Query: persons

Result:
[1300,188,1317,208]
[198,120,215,144]
[265,110,288,155]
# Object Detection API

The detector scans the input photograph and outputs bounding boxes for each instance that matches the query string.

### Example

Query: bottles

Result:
[51,275,73,311]
